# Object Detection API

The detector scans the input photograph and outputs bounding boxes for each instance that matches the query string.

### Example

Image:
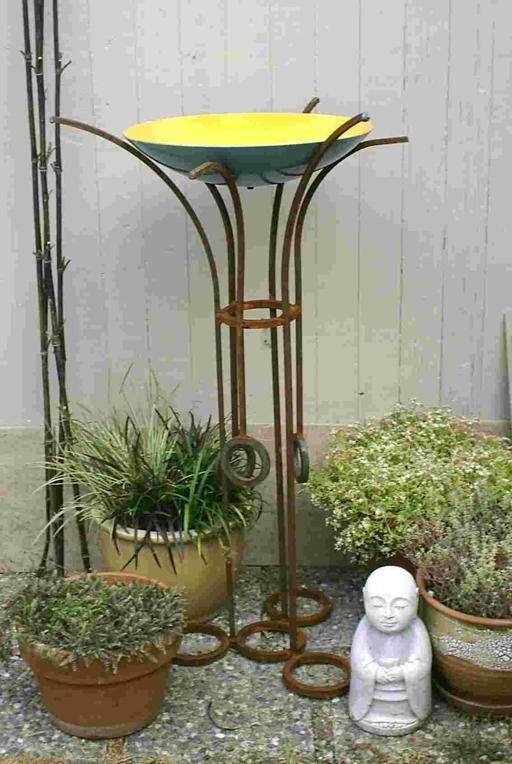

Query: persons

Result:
[347,566,435,737]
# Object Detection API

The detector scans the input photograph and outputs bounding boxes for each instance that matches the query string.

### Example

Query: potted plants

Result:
[24,355,277,626]
[297,397,510,717]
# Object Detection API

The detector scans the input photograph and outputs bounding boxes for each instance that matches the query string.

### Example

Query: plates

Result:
[121,110,375,190]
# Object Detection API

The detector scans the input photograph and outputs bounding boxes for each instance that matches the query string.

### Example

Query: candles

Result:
[1,547,191,741]
